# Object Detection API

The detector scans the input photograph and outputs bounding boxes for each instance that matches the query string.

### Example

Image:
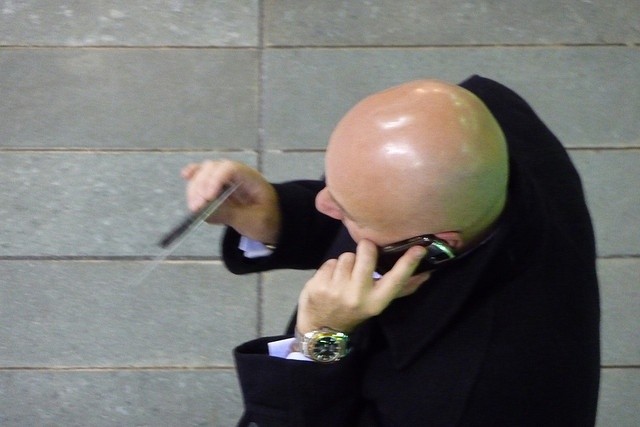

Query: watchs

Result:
[288,324,354,363]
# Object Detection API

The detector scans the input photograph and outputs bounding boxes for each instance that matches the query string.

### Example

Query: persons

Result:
[181,72,602,427]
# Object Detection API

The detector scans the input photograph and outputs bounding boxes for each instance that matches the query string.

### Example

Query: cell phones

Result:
[375,233,457,276]
